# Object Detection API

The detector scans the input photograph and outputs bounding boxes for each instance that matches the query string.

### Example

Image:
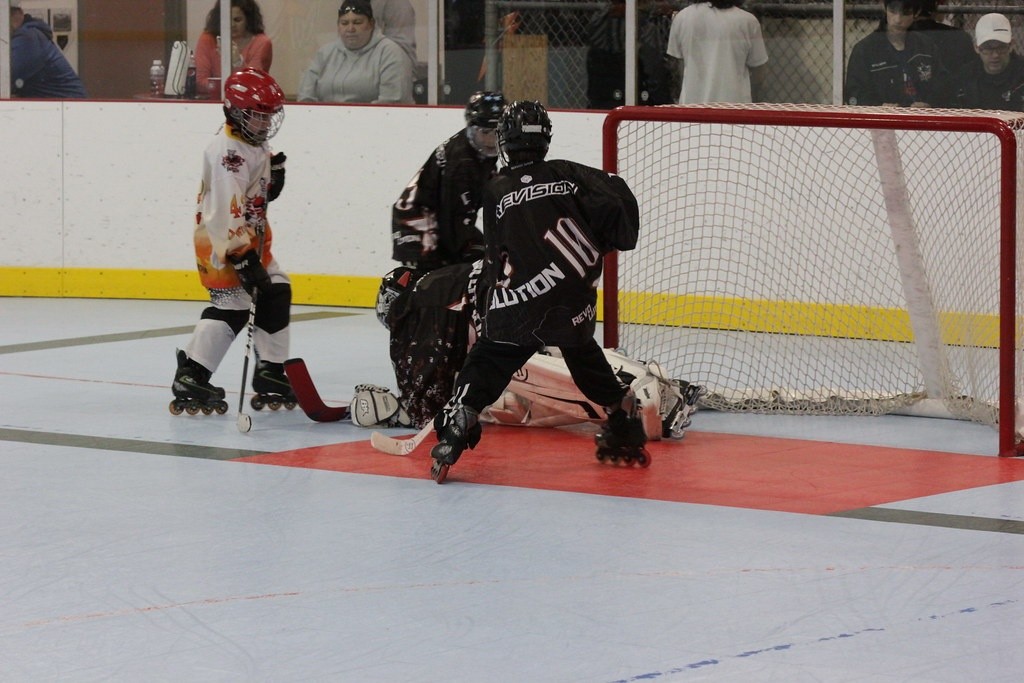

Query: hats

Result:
[975,13,1013,49]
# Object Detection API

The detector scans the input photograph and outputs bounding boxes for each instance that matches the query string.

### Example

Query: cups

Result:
[207,78,221,100]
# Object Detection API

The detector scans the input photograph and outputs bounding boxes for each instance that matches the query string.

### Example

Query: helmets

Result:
[465,92,506,128]
[378,266,419,326]
[223,67,287,143]
[496,101,553,170]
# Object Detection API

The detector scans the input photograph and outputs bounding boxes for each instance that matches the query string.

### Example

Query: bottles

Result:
[149,59,166,98]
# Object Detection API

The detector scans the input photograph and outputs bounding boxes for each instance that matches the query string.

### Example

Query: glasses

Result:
[340,6,373,20]
[976,44,1011,55]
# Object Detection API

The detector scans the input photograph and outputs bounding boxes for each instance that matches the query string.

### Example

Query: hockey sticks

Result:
[283,358,351,422]
[238,201,268,433]
[371,419,433,455]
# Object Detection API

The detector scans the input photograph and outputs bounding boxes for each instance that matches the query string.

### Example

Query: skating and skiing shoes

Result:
[250,344,300,411]
[594,413,651,467]
[427,405,482,483]
[660,378,708,437]
[170,348,228,415]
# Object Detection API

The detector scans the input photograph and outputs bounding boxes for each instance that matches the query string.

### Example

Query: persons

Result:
[431,101,651,484]
[169,67,300,416]
[9,7,90,98]
[195,0,273,101]
[844,0,945,108]
[295,1,416,103]
[351,262,707,440]
[909,14,1024,112]
[666,0,769,105]
[391,93,511,430]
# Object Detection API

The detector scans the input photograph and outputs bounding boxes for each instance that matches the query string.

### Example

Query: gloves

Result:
[268,152,286,201]
[225,248,272,299]
[421,246,449,275]
